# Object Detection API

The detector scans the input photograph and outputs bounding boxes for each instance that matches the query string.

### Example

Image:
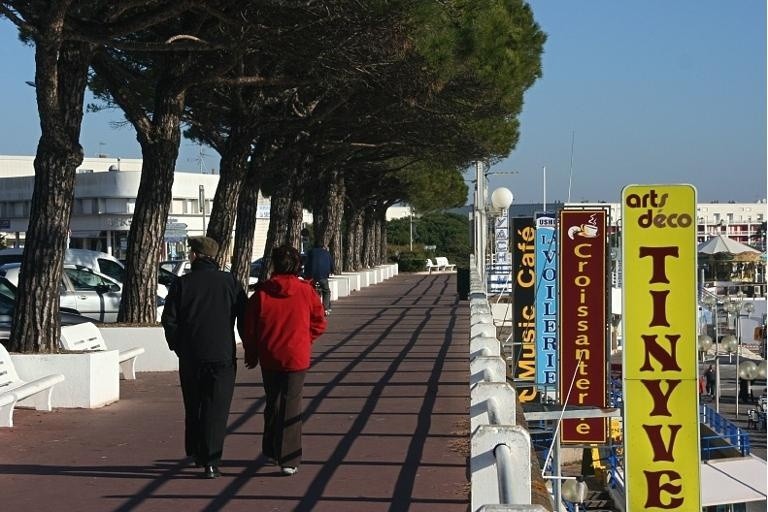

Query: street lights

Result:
[698,294,767,420]
[474,186,514,284]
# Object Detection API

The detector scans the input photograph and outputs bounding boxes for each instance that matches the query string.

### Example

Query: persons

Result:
[303,238,336,318]
[160,236,259,479]
[702,364,717,399]
[243,246,327,476]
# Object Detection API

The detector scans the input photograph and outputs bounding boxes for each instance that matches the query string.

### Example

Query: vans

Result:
[0,248,168,299]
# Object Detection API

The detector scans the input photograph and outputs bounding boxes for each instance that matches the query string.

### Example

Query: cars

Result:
[0,270,104,346]
[160,259,259,294]
[0,262,167,324]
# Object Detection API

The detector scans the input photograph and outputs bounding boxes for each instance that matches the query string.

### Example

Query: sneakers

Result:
[265,456,299,475]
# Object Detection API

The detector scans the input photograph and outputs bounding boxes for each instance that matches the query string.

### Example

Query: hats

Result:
[187,236,219,258]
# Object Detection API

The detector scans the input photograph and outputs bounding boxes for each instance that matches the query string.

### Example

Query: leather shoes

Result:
[205,464,221,479]
[186,456,203,468]
